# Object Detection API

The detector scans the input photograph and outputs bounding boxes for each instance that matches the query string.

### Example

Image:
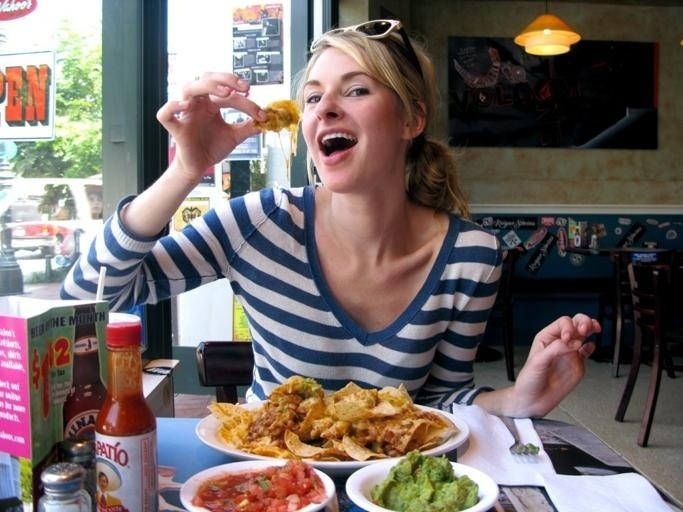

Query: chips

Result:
[207,380,454,462]
[266,100,300,125]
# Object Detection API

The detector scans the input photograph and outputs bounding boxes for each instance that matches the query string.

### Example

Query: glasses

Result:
[310,19,423,81]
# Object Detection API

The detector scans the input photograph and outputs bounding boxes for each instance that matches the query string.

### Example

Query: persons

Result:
[96,472,121,508]
[60,27,601,420]
[88,188,103,220]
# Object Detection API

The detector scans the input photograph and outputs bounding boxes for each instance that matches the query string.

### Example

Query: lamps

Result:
[513,0,583,57]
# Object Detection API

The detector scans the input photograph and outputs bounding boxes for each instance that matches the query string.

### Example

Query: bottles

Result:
[37,461,93,512]
[63,305,109,471]
[94,321,159,512]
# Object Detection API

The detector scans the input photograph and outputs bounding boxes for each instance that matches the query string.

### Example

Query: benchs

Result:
[465,214,683,345]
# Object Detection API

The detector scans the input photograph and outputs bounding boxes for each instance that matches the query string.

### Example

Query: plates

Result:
[180,460,336,512]
[345,456,499,512]
[195,399,469,477]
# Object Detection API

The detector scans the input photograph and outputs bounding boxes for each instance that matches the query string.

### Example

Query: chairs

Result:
[615,263,682,447]
[482,249,521,381]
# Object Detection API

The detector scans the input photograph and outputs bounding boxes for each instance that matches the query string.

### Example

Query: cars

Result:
[0,177,103,281]
